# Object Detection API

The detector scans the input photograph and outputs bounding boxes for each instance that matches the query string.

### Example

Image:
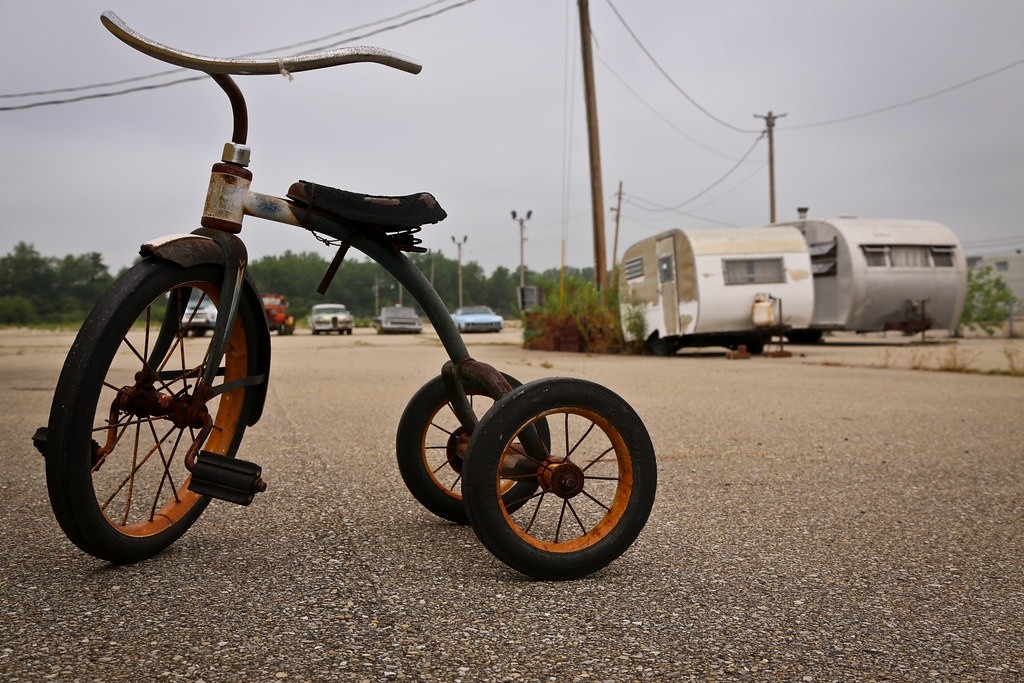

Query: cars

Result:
[309,304,355,335]
[450,305,504,333]
[260,292,295,334]
[177,288,218,338]
[376,306,423,334]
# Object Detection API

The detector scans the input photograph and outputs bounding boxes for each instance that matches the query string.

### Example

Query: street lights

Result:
[510,210,533,288]
[451,235,468,309]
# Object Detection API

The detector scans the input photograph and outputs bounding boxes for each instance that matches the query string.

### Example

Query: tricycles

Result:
[33,8,659,584]
